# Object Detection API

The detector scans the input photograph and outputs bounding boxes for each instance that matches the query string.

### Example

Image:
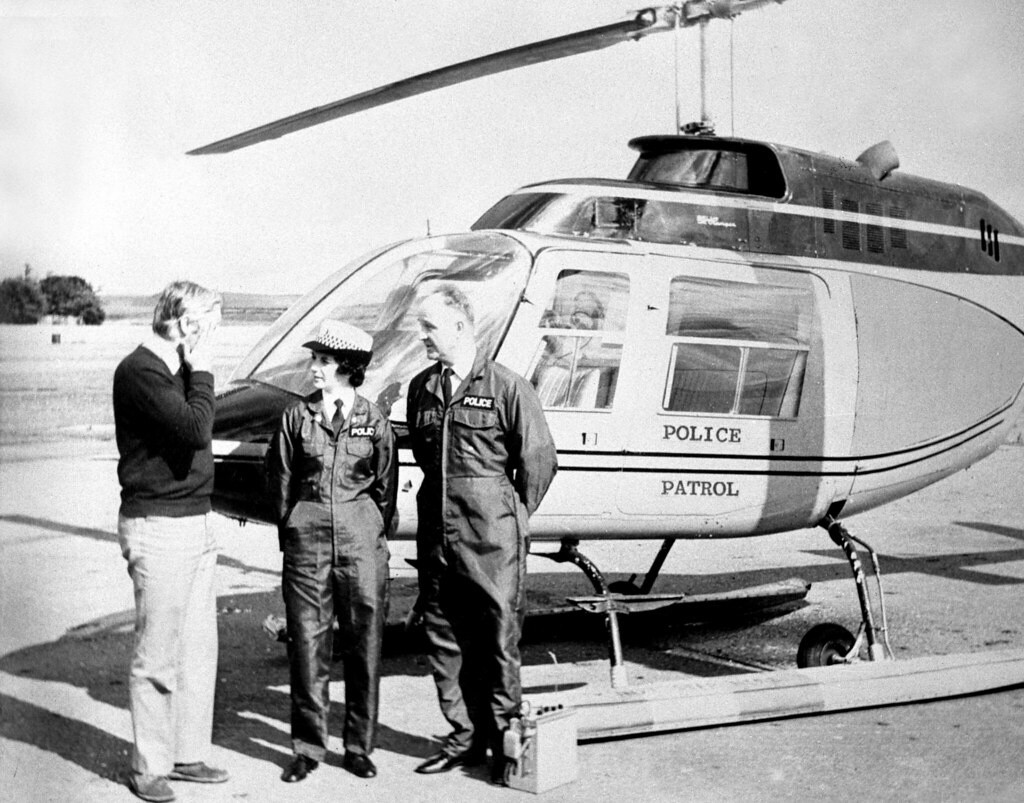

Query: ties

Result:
[443,368,455,410]
[331,399,345,436]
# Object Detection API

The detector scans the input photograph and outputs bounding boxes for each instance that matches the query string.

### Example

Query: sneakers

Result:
[129,774,175,802]
[168,762,230,783]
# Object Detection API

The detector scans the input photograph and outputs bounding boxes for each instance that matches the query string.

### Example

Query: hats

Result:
[301,319,373,352]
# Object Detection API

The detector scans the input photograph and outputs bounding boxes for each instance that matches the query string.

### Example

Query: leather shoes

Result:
[342,749,376,778]
[414,749,486,773]
[490,754,509,785]
[281,753,318,782]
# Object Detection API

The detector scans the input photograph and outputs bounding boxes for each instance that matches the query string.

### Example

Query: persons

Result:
[111,279,230,803]
[260,318,402,782]
[406,283,559,781]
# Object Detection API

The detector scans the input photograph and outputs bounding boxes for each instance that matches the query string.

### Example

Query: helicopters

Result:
[185,0,1023,746]
